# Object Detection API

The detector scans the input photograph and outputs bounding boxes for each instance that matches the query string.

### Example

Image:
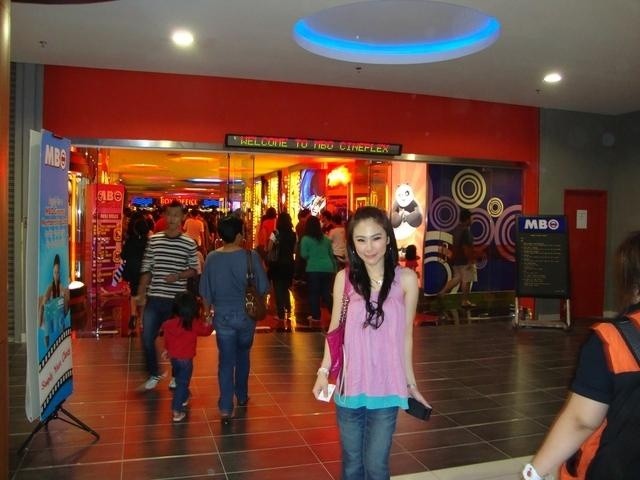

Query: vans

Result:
[284,318,291,334]
[276,319,285,336]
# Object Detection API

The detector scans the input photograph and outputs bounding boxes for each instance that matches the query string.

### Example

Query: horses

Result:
[404,397,432,421]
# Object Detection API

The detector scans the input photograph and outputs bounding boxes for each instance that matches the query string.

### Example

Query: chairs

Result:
[462,303,476,307]
[173,409,187,421]
[168,377,177,389]
[144,370,168,389]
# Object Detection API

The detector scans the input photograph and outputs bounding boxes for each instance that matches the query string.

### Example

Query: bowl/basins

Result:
[371,277,384,286]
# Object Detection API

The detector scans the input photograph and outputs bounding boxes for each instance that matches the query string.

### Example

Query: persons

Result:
[183,208,220,256]
[38,254,71,346]
[138,201,200,406]
[437,210,481,307]
[119,206,170,334]
[517,228,640,480]
[156,292,214,423]
[257,206,350,320]
[311,206,434,480]
[199,216,269,418]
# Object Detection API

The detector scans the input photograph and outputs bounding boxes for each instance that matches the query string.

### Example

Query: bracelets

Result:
[176,273,179,280]
[406,382,417,389]
[522,464,541,480]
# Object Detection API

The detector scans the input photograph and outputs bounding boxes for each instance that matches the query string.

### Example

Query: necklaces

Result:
[371,277,384,286]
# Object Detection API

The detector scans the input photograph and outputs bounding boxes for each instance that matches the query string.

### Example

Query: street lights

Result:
[245,283,264,321]
[321,323,345,385]
[463,263,478,282]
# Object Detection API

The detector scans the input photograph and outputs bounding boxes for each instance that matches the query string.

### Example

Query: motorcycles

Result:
[17,406,100,454]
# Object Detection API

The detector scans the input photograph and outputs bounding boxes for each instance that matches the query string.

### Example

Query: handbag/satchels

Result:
[522,464,541,480]
[406,382,417,389]
